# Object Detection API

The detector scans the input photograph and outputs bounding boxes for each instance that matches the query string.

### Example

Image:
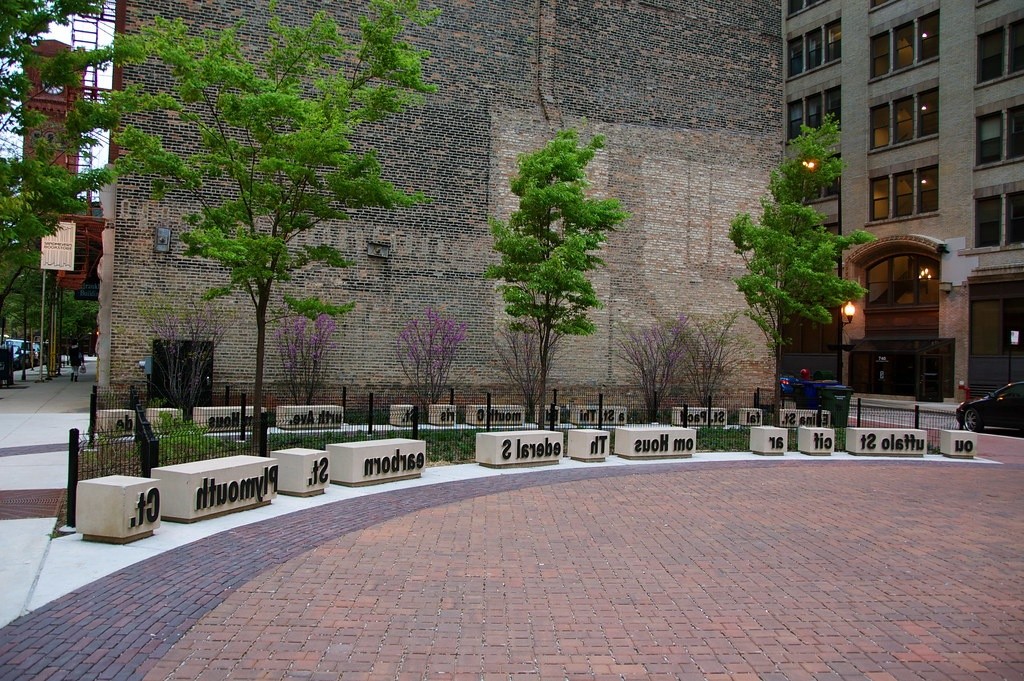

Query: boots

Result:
[70,373,74,381]
[75,376,78,382]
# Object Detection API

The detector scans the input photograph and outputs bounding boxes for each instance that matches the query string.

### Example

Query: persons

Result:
[800,368,811,380]
[68,339,84,382]
[813,371,826,381]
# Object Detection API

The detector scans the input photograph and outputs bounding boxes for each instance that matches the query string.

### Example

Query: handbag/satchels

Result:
[80,365,86,373]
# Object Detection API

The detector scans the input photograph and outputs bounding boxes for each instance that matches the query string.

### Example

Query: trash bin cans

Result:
[792,379,855,427]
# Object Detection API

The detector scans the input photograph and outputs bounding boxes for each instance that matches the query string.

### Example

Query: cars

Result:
[0,339,40,375]
[774,368,800,398]
[954,380,1024,432]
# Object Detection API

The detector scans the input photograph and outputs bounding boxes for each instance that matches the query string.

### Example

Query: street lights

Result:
[20,283,30,380]
